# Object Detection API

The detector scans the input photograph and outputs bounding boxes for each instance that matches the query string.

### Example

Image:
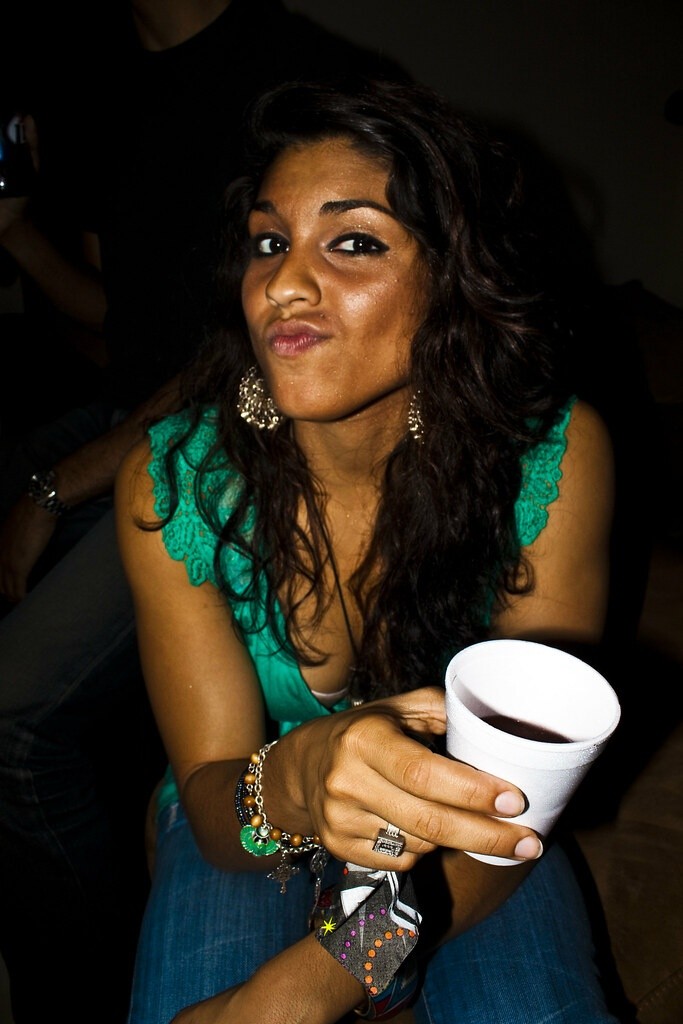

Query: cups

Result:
[445,640,622,866]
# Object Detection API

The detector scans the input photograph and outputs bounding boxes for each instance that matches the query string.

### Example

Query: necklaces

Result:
[308,483,363,664]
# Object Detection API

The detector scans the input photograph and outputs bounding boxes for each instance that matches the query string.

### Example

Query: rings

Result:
[372,824,405,857]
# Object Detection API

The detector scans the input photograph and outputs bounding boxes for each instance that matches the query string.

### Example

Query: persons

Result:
[0,0,401,888]
[113,82,617,1024]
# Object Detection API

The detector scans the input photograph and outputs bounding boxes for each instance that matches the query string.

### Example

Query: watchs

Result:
[26,470,70,516]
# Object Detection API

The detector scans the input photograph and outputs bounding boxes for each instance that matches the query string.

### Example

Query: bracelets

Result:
[235,741,327,924]
